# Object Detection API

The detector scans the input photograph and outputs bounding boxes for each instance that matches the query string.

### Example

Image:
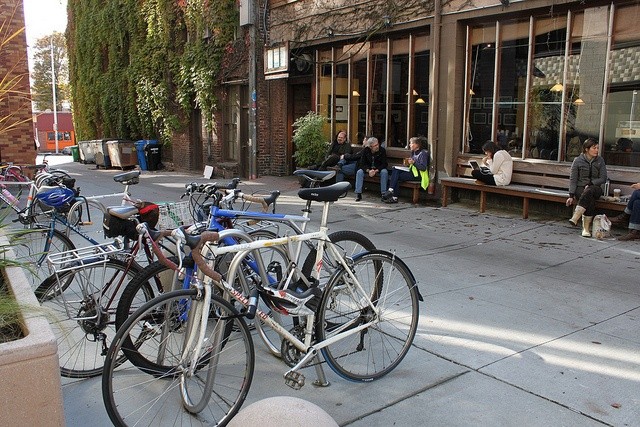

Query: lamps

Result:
[352,90,361,96]
[415,98,425,103]
[573,98,586,105]
[550,81,564,91]
[469,89,475,94]
[406,89,419,95]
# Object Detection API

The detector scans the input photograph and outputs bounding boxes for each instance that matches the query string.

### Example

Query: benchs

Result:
[440,152,639,219]
[328,141,428,202]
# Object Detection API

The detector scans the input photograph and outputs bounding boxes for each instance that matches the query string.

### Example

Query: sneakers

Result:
[384,197,398,203]
[382,190,394,196]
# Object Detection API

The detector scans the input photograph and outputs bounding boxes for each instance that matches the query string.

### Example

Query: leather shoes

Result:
[607,212,631,224]
[355,196,362,201]
[619,229,640,241]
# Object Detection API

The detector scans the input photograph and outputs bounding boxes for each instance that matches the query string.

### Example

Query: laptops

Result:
[469,160,490,172]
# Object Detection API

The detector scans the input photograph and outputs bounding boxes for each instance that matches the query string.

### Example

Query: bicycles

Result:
[33,171,294,377]
[116,169,383,377]
[0,164,82,232]
[0,152,71,209]
[214,186,296,332]
[10,170,184,301]
[103,180,423,426]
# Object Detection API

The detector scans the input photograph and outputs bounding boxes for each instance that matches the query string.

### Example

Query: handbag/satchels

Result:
[592,214,612,238]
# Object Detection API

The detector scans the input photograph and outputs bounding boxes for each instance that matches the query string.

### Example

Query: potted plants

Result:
[291,104,330,186]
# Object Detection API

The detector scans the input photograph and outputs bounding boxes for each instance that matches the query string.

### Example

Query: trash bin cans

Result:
[70,145,79,162]
[134,139,159,171]
[106,140,138,170]
[143,143,163,171]
[78,141,95,164]
[92,138,122,169]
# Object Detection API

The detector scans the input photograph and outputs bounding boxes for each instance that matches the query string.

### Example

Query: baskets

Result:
[157,200,190,232]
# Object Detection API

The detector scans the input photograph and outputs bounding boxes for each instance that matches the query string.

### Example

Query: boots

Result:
[569,204,586,225]
[582,214,594,236]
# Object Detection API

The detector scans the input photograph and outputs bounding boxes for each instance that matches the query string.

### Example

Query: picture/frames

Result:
[488,112,501,125]
[421,111,428,123]
[500,95,512,108]
[359,111,366,122]
[328,94,351,122]
[375,110,385,123]
[514,97,518,109]
[471,97,482,109]
[483,97,493,109]
[503,113,516,125]
[474,112,487,124]
[392,109,402,122]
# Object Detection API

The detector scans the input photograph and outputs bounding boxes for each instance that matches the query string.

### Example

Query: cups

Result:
[613,188,621,197]
[404,158,410,166]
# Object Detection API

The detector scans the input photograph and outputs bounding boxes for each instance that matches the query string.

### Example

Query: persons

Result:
[565,137,607,237]
[605,182,640,241]
[299,131,353,188]
[354,137,391,204]
[470,141,513,187]
[327,136,370,182]
[614,138,633,152]
[381,137,429,203]
